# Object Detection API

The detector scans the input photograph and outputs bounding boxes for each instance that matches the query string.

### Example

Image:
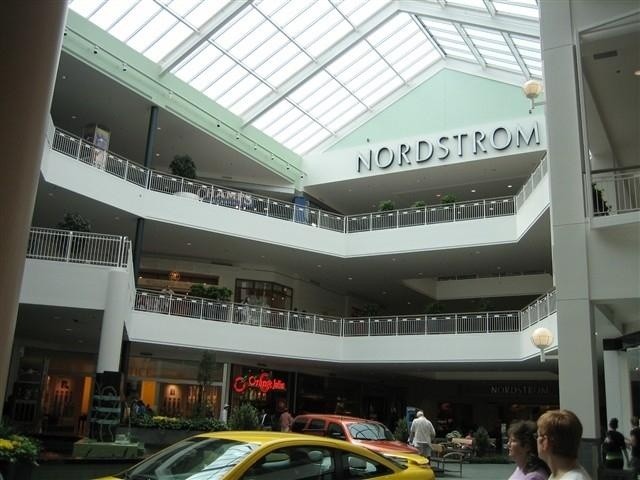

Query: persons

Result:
[253,402,294,433]
[240,296,250,325]
[503,418,552,480]
[235,299,244,322]
[600,417,629,470]
[218,403,230,424]
[622,414,640,470]
[135,400,146,419]
[146,404,152,411]
[535,409,594,480]
[406,409,436,465]
[197,183,251,211]
[162,285,174,294]
[130,396,139,418]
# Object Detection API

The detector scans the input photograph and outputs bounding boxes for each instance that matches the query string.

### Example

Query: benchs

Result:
[428,436,475,478]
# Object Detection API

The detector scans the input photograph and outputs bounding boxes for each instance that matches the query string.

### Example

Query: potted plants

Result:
[168,152,197,201]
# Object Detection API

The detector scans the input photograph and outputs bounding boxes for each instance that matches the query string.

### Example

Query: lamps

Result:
[523,75,545,111]
[531,328,558,362]
